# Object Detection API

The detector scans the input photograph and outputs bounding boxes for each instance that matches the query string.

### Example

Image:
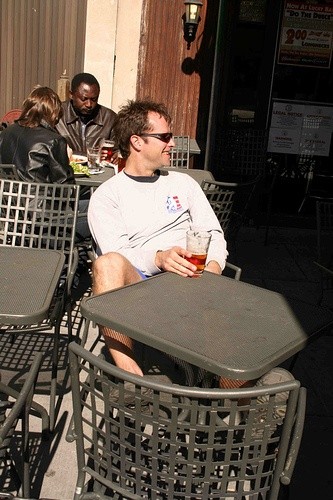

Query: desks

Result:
[170,137,200,154]
[75,167,115,187]
[162,168,216,189]
[1,246,65,325]
[80,268,306,380]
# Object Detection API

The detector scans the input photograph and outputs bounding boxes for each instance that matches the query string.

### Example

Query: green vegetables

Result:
[69,161,91,177]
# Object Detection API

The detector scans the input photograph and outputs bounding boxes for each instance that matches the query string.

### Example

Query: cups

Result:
[185,229,212,278]
[118,149,125,173]
[86,147,101,173]
[102,140,115,164]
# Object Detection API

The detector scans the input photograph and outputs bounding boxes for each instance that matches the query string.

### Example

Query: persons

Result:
[1,87,91,312]
[87,100,229,408]
[55,72,119,165]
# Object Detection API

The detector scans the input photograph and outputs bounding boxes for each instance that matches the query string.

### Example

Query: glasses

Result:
[132,132,174,144]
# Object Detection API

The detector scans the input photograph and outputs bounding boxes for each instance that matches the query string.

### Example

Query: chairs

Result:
[0,112,333,500]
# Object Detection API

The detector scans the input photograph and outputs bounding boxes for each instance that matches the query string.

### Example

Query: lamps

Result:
[181,0,203,50]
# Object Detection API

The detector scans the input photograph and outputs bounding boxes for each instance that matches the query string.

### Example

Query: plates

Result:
[72,154,88,164]
[74,173,86,177]
